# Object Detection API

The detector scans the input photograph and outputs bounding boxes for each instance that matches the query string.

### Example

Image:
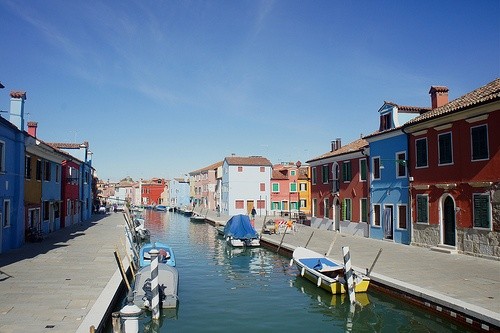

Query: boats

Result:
[134,263,178,310]
[100,203,260,248]
[139,242,176,268]
[292,247,370,294]
